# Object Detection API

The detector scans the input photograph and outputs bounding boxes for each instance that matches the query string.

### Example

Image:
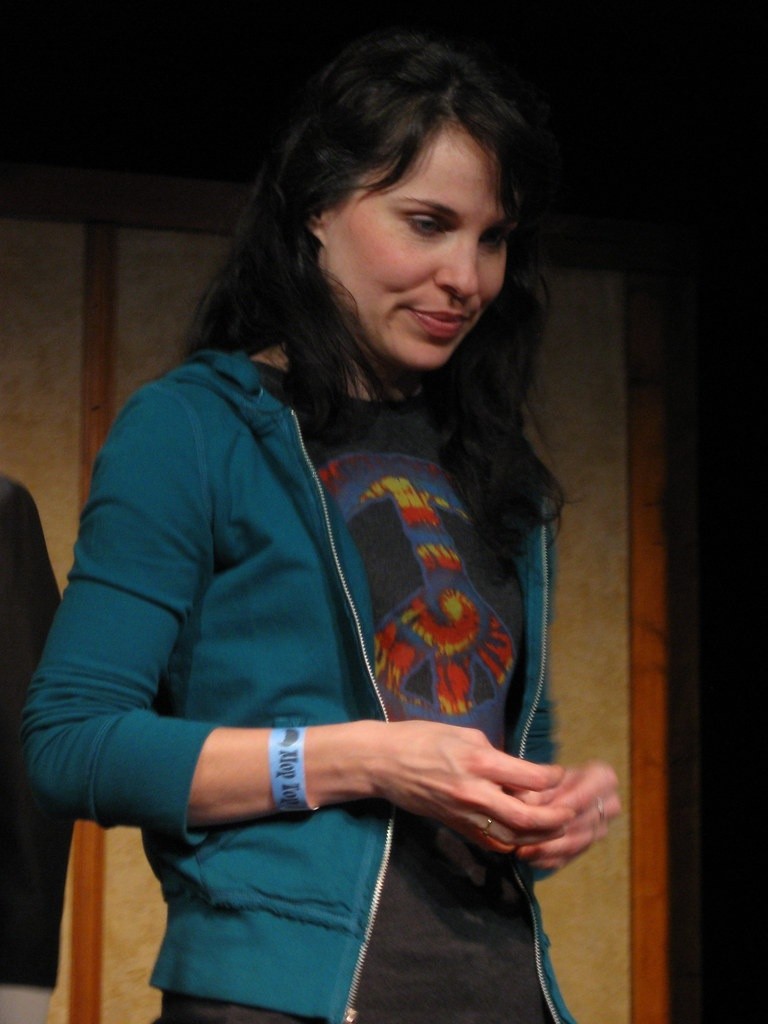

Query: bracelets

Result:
[268,727,320,811]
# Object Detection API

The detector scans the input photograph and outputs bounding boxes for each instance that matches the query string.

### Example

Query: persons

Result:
[18,33,620,1024]
[0,479,76,1024]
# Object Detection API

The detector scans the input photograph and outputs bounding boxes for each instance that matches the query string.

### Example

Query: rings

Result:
[599,804,605,822]
[483,818,493,835]
